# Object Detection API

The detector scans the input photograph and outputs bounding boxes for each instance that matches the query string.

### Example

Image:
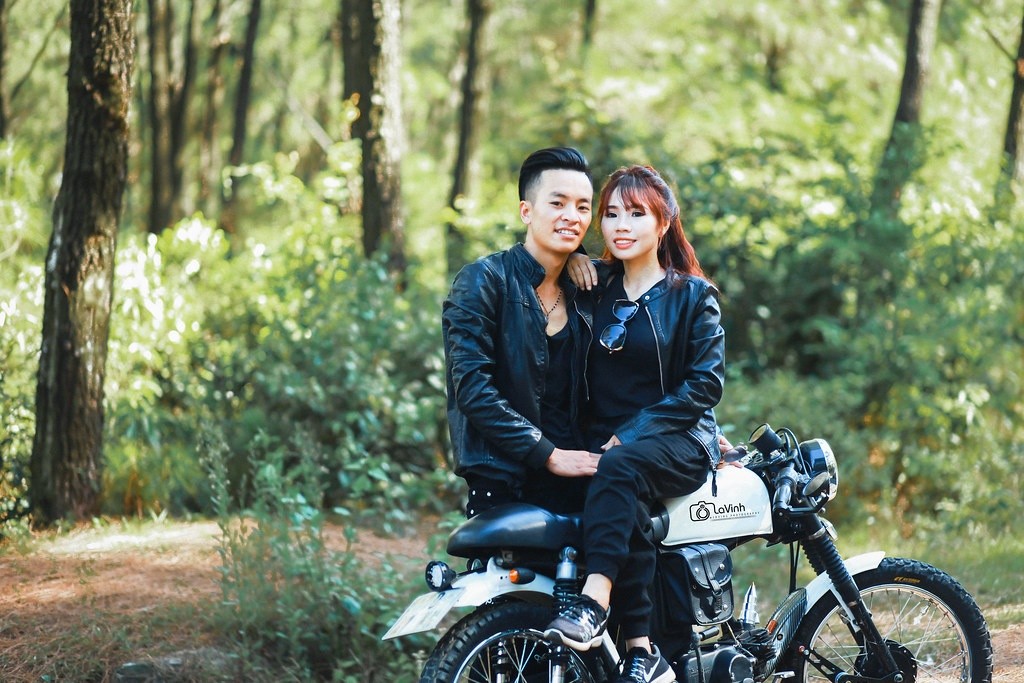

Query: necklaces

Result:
[535,288,562,328]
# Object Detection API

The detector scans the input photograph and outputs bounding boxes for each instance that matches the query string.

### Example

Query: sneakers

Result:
[617,645,676,683]
[543,594,610,652]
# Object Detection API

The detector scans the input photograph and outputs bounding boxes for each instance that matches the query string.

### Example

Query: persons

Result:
[543,164,725,683]
[442,147,743,683]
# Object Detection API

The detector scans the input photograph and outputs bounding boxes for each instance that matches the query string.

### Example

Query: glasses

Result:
[599,298,640,353]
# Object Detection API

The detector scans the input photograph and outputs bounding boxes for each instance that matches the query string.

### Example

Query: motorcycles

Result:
[382,424,995,683]
